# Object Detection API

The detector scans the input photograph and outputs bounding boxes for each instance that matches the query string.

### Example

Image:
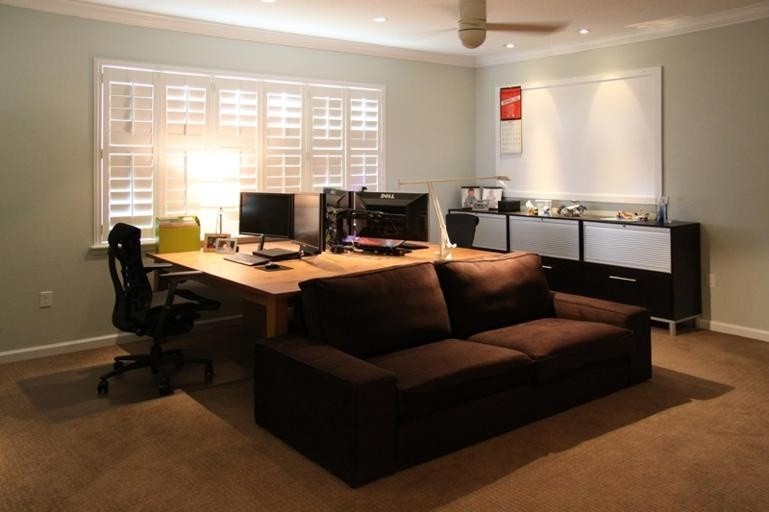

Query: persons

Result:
[464,189,478,208]
[486,190,496,208]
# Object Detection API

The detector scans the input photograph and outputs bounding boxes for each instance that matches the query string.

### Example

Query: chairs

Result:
[96,222,222,396]
[445,213,479,249]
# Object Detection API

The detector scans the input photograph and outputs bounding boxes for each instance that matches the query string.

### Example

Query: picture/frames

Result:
[481,187,503,210]
[460,186,480,209]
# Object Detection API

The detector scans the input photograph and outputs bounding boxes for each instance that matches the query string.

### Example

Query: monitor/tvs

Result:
[323,187,354,237]
[354,191,429,242]
[238,192,292,250]
[292,192,326,254]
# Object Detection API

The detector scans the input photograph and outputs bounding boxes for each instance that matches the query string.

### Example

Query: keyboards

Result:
[223,252,270,266]
[400,242,429,250]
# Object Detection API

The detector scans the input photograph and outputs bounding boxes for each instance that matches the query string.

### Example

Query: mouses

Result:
[265,263,280,269]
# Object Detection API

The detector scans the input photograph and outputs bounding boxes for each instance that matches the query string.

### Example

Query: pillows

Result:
[433,251,557,338]
[298,261,452,359]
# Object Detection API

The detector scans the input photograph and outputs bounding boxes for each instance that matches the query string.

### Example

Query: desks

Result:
[145,240,436,338]
[404,242,505,262]
[448,207,704,336]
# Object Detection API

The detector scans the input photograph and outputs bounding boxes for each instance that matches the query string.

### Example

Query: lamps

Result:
[399,172,511,261]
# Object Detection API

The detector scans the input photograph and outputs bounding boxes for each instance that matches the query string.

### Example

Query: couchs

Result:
[253,290,652,488]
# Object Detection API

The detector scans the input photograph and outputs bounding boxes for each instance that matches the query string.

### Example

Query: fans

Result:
[404,1,568,49]
